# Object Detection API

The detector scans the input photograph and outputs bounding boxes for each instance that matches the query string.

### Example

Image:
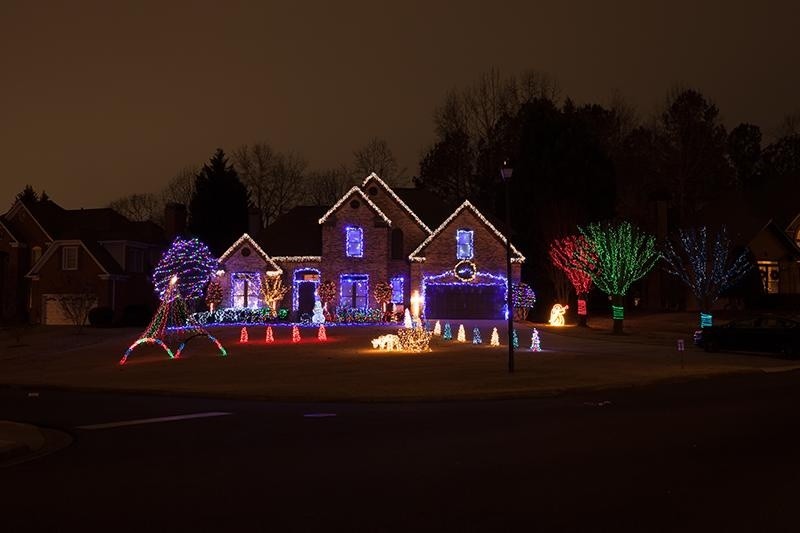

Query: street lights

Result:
[500,158,516,376]
